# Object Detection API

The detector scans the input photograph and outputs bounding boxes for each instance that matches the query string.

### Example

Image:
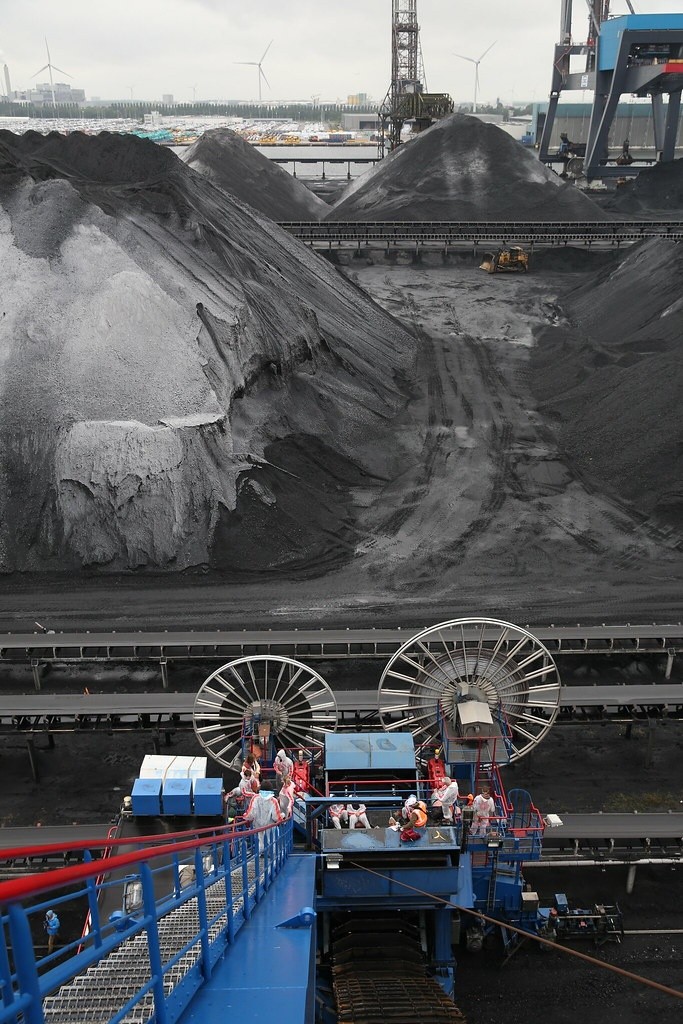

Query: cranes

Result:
[374,0,456,163]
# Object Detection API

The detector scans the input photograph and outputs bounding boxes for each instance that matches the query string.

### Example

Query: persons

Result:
[328,803,373,830]
[399,777,500,837]
[223,749,297,859]
[44,909,60,955]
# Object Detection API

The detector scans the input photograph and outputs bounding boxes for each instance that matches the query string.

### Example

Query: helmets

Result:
[407,797,416,806]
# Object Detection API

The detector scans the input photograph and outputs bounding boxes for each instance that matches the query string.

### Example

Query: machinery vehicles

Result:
[479,244,530,274]
